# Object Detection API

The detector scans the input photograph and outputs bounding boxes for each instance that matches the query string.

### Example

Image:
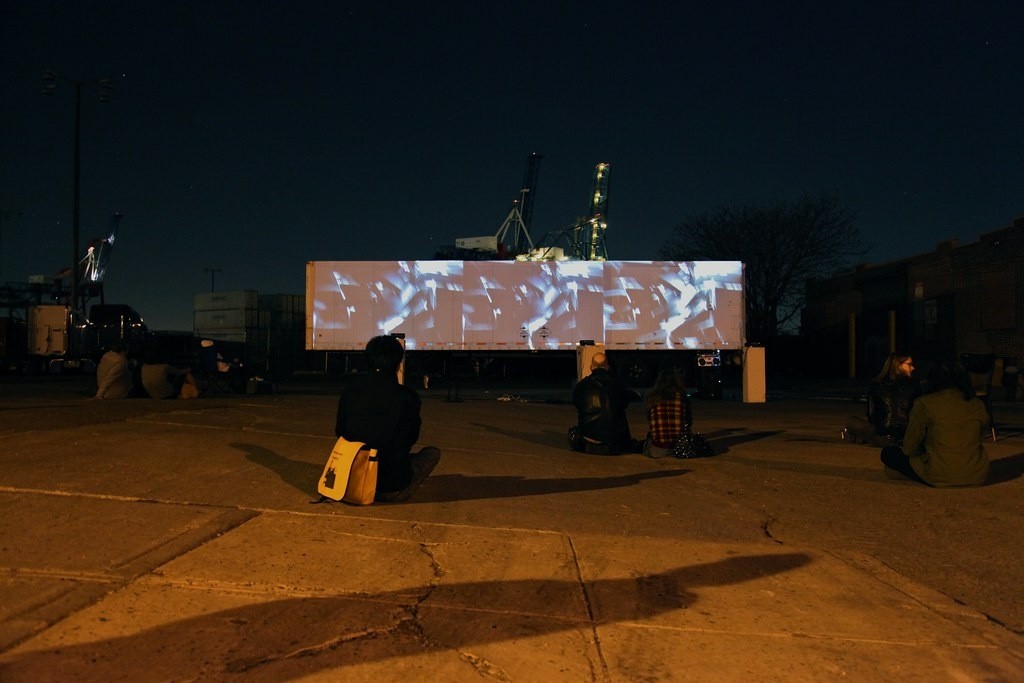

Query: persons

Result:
[336,336,441,503]
[880,370,990,488]
[636,369,706,458]
[923,360,993,426]
[1003,360,1019,401]
[568,352,636,456]
[88,340,199,401]
[846,351,921,447]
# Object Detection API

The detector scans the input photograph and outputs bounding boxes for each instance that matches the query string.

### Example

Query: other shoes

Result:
[885,466,910,480]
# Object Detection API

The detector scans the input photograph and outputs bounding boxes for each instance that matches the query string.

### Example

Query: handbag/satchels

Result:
[871,434,895,447]
[317,436,377,505]
[673,432,716,459]
